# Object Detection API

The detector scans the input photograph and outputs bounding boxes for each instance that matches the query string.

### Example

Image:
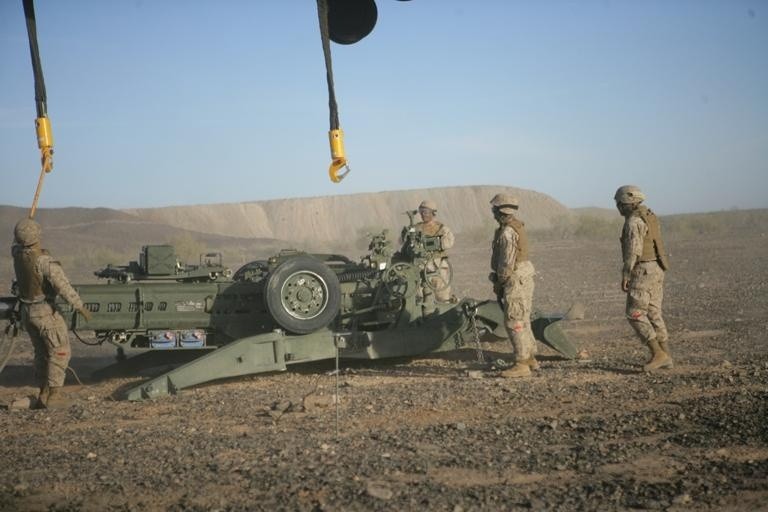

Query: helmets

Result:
[614,186,645,204]
[14,219,42,247]
[489,193,519,215]
[420,201,438,210]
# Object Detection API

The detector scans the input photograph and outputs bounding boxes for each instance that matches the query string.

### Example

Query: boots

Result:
[37,385,77,409]
[501,355,540,378]
[642,339,674,371]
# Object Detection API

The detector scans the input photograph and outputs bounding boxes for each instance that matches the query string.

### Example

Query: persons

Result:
[412,198,455,305]
[11,217,92,410]
[490,192,541,378]
[614,184,674,372]
